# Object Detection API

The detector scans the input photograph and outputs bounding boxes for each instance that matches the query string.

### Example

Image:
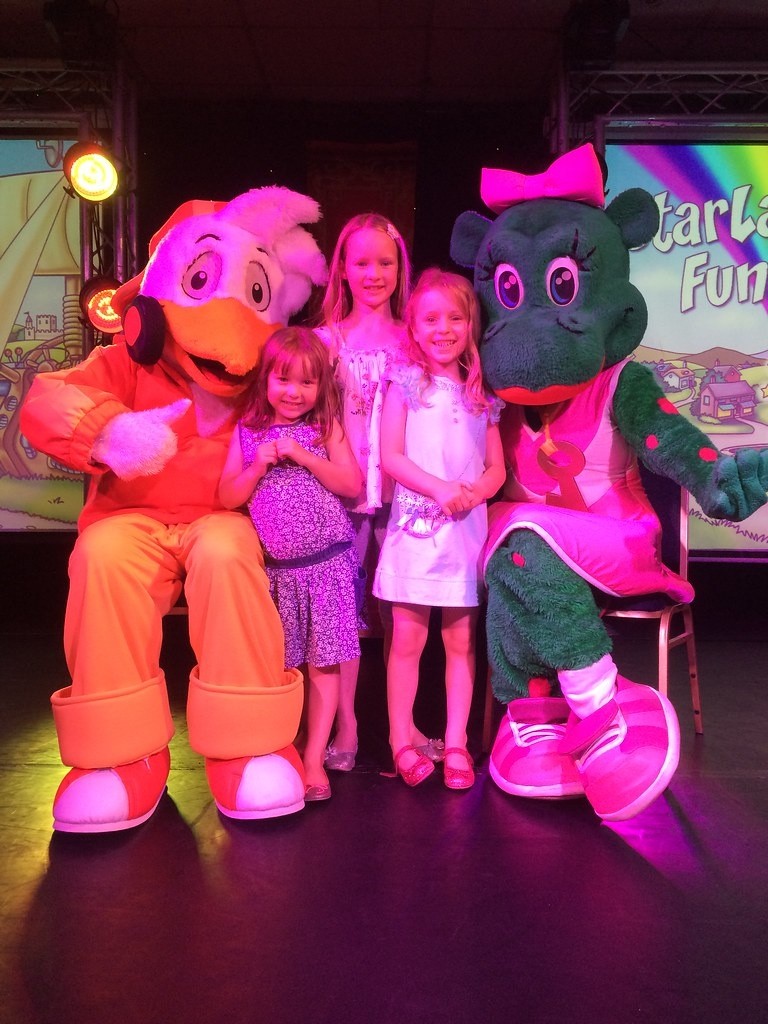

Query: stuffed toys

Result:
[19,185,330,835]
[446,140,768,822]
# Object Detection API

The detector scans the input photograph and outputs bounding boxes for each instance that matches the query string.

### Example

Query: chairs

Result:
[478,460,705,753]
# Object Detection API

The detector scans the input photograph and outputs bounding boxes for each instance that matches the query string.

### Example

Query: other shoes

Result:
[393,743,436,788]
[443,746,476,790]
[323,735,359,772]
[302,777,332,802]
[414,738,446,763]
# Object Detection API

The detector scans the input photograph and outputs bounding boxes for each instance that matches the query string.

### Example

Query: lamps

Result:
[79,273,125,334]
[61,142,120,203]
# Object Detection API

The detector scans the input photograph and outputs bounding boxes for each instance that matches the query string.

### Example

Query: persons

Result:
[220,213,507,802]
